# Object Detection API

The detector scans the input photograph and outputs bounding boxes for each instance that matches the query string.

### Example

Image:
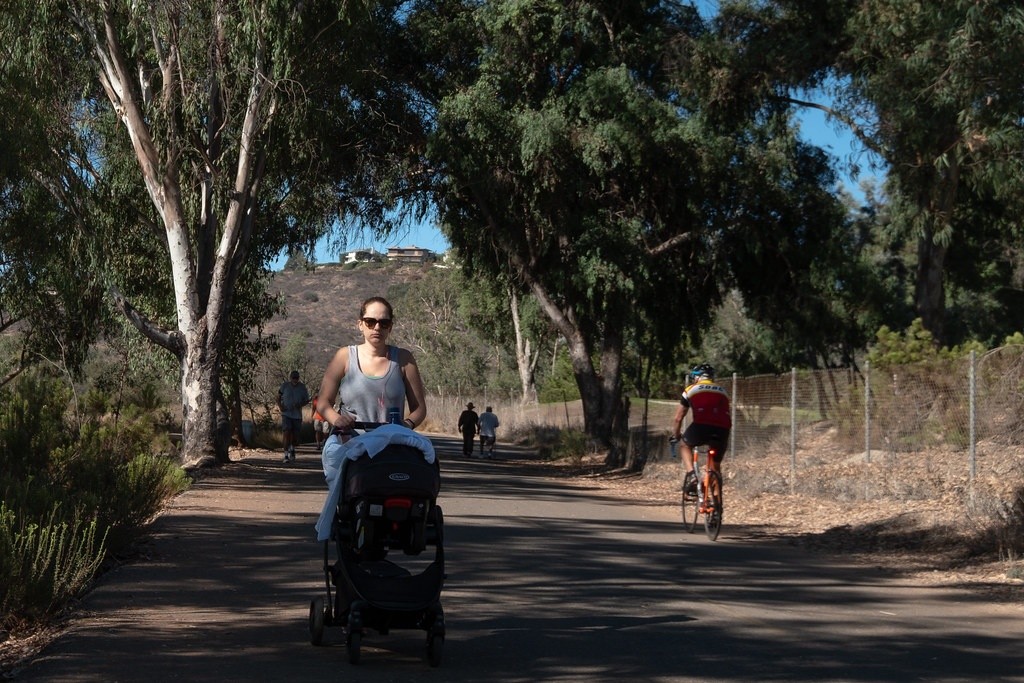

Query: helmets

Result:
[691,364,715,377]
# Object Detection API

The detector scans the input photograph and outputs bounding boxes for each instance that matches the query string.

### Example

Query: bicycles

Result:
[670,434,725,541]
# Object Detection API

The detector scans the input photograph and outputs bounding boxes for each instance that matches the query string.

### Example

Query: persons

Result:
[308,390,325,449]
[669,363,733,515]
[457,402,481,458]
[277,370,311,465]
[314,296,431,639]
[477,406,499,460]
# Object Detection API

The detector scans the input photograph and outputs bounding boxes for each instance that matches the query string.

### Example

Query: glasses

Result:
[292,376,299,379]
[361,318,392,328]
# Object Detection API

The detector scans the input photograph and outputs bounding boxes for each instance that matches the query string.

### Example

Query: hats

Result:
[464,402,475,408]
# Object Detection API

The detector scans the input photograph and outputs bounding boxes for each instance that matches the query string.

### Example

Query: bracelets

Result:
[405,417,416,429]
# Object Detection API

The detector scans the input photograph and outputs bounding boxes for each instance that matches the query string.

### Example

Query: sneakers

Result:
[283,456,290,463]
[290,449,296,461]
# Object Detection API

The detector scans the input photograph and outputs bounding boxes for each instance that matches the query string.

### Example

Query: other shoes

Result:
[488,453,492,459]
[686,477,698,494]
[479,453,484,457]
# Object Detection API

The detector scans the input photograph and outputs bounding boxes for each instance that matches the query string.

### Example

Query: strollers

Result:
[310,422,450,666]
